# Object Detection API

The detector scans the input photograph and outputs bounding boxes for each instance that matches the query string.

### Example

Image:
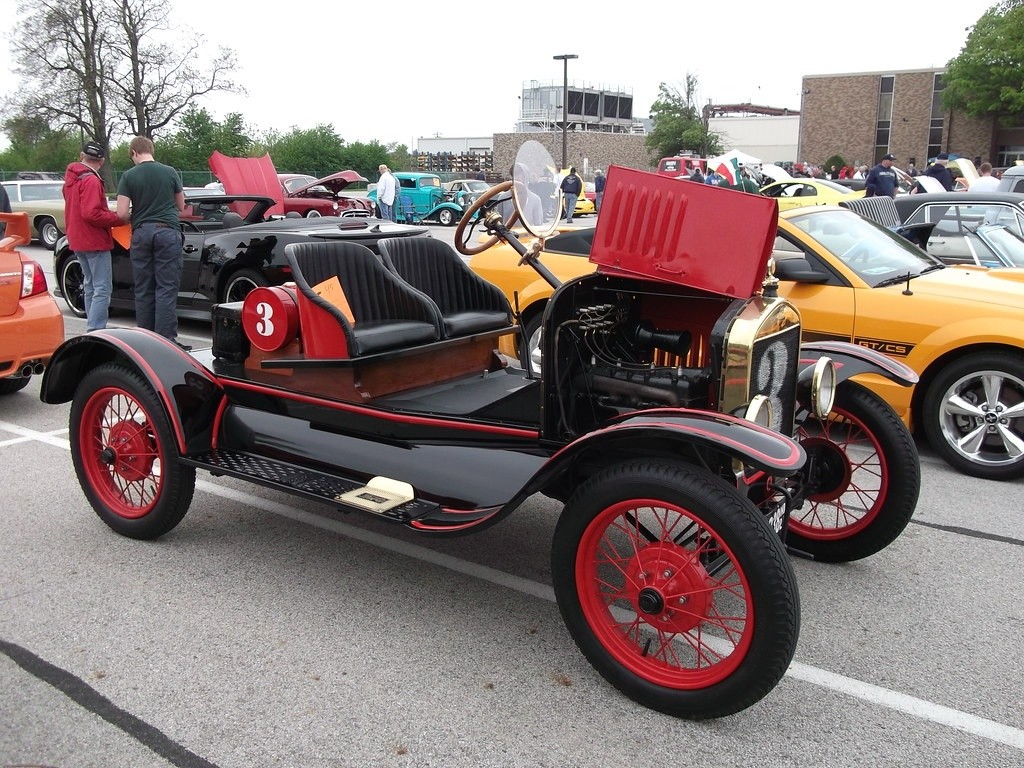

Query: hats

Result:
[937,153,948,160]
[883,153,897,160]
[84,141,105,158]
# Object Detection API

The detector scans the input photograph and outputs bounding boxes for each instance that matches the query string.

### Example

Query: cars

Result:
[39,139,921,721]
[657,155,1024,268]
[5,170,600,252]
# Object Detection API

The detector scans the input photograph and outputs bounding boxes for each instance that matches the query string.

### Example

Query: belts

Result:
[139,222,170,228]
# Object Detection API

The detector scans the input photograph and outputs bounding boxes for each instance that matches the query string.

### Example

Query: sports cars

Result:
[53,194,433,325]
[1,210,65,396]
[469,199,1024,479]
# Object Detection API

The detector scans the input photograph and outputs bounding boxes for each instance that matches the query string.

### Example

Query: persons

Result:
[737,161,766,187]
[116,135,193,351]
[705,170,722,187]
[905,161,918,177]
[967,161,1003,193]
[803,160,869,180]
[559,166,582,223]
[909,150,958,195]
[376,164,400,223]
[496,163,543,227]
[689,169,705,183]
[62,140,129,331]
[0,182,11,241]
[864,154,900,201]
[594,169,606,215]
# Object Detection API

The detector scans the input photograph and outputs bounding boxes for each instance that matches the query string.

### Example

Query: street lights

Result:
[552,54,580,170]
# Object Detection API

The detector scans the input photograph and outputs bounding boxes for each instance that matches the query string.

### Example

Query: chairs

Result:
[285,240,439,360]
[377,236,515,343]
[223,212,245,229]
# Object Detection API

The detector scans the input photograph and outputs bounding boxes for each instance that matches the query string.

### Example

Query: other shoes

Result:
[170,339,193,351]
[567,219,573,223]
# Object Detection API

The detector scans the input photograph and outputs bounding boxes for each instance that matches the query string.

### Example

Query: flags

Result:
[715,157,742,187]
